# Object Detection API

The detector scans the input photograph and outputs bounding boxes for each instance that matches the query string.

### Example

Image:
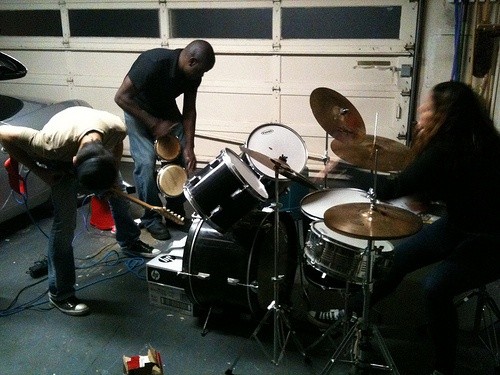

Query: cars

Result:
[1,52,93,228]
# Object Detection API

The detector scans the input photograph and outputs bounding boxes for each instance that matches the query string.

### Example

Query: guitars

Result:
[32,156,185,226]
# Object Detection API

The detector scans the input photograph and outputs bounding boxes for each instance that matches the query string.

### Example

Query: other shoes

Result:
[147,222,171,241]
[166,216,191,232]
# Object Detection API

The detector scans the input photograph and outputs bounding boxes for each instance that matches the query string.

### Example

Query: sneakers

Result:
[306,309,347,326]
[121,241,162,258]
[47,296,90,316]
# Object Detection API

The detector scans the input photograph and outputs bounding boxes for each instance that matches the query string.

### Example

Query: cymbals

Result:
[0,95,24,121]
[323,203,423,241]
[310,87,367,143]
[239,146,319,191]
[332,136,417,171]
[0,51,27,80]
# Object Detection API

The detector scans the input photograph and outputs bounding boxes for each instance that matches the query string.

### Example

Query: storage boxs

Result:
[145,253,187,290]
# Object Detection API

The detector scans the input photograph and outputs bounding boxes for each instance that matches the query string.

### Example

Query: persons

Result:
[114,40,215,240]
[0,106,161,315]
[306,81,500,331]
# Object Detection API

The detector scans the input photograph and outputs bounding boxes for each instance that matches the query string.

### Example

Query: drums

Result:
[182,212,300,325]
[183,149,269,234]
[154,134,182,162]
[304,221,395,286]
[299,187,381,291]
[154,163,188,197]
[239,122,308,200]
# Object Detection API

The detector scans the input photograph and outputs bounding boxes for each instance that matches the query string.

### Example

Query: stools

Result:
[413,284,500,375]
